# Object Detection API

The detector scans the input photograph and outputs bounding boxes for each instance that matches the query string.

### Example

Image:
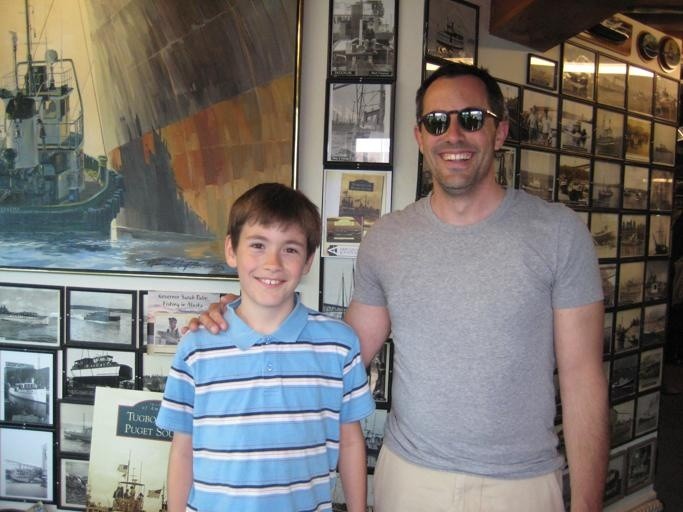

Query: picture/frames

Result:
[65,287,138,349]
[58,459,88,512]
[1,429,58,506]
[327,1,399,78]
[1,0,304,279]
[1,283,63,349]
[333,337,392,512]
[420,0,682,509]
[139,352,177,394]
[318,256,355,318]
[320,170,392,257]
[139,290,223,352]
[89,388,175,511]
[322,80,396,167]
[62,351,139,404]
[0,346,58,428]
[58,401,91,460]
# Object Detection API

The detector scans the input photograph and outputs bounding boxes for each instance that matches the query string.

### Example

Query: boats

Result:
[7,381,48,405]
[437,16,464,51]
[71,355,133,382]
[113,451,145,512]
[84,310,121,323]
[64,427,93,444]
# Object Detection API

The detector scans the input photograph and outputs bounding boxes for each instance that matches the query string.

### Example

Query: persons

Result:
[182,63,611,512]
[167,318,180,342]
[527,105,539,146]
[155,182,376,512]
[540,108,550,147]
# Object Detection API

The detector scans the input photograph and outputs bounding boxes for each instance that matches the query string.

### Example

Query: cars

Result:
[327,218,362,243]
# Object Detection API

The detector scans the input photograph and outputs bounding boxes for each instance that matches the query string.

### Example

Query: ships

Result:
[1,305,48,325]
[116,113,219,243]
[1,1,127,233]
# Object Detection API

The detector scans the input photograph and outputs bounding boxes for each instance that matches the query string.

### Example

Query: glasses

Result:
[419,106,502,136]
